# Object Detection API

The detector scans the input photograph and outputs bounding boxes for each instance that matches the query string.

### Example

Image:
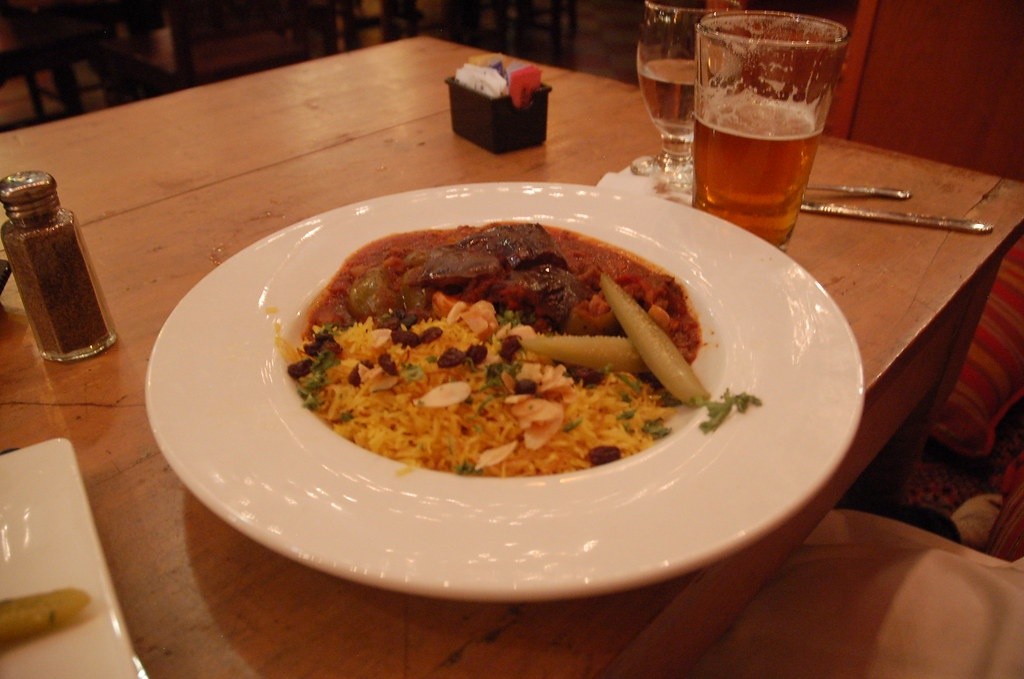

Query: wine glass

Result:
[632,0,748,207]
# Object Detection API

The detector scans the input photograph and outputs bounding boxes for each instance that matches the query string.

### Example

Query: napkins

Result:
[595,160,693,205]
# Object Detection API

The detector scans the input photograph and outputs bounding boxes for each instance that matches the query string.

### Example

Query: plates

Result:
[143,180,866,605]
[0,436,150,679]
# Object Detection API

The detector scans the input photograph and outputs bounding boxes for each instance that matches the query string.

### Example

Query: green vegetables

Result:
[294,311,761,477]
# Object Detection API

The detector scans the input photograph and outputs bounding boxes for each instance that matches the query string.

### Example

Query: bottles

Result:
[0,170,117,363]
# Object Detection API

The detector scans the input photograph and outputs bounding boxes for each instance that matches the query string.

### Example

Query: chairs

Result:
[26,0,578,121]
[698,509,1024,679]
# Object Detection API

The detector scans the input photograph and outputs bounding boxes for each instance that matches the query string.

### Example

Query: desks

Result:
[0,37,1024,679]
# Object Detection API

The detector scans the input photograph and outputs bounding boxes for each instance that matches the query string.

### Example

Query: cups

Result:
[693,12,852,255]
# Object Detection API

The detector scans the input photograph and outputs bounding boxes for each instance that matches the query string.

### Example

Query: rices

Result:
[264,308,664,480]
